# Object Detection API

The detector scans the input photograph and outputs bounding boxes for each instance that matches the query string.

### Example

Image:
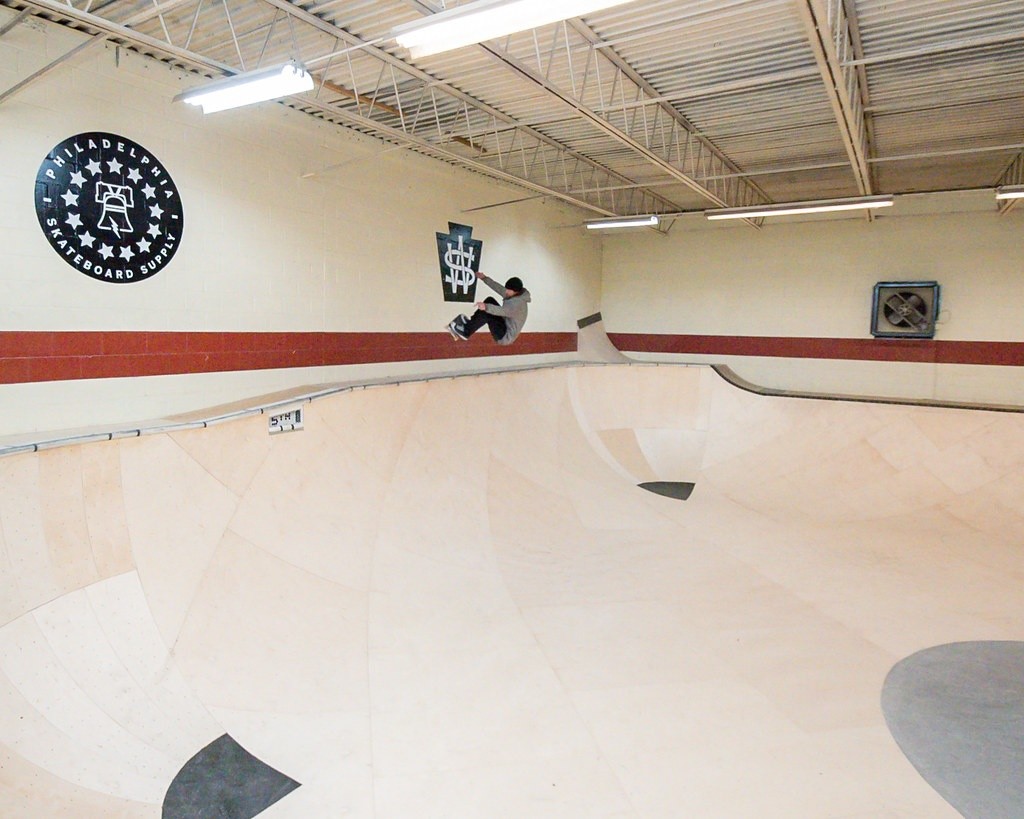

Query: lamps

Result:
[582,215,660,228]
[703,193,895,220]
[995,185,1024,199]
[171,7,315,116]
[383,1,637,60]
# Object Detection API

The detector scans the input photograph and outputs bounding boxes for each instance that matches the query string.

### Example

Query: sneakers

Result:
[461,314,470,325]
[450,322,468,340]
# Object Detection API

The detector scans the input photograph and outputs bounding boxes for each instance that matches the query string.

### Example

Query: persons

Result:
[448,271,532,347]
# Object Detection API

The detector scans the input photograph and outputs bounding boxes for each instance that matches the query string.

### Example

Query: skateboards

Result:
[445,313,465,342]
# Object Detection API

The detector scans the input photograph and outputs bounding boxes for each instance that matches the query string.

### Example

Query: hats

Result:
[505,277,522,292]
